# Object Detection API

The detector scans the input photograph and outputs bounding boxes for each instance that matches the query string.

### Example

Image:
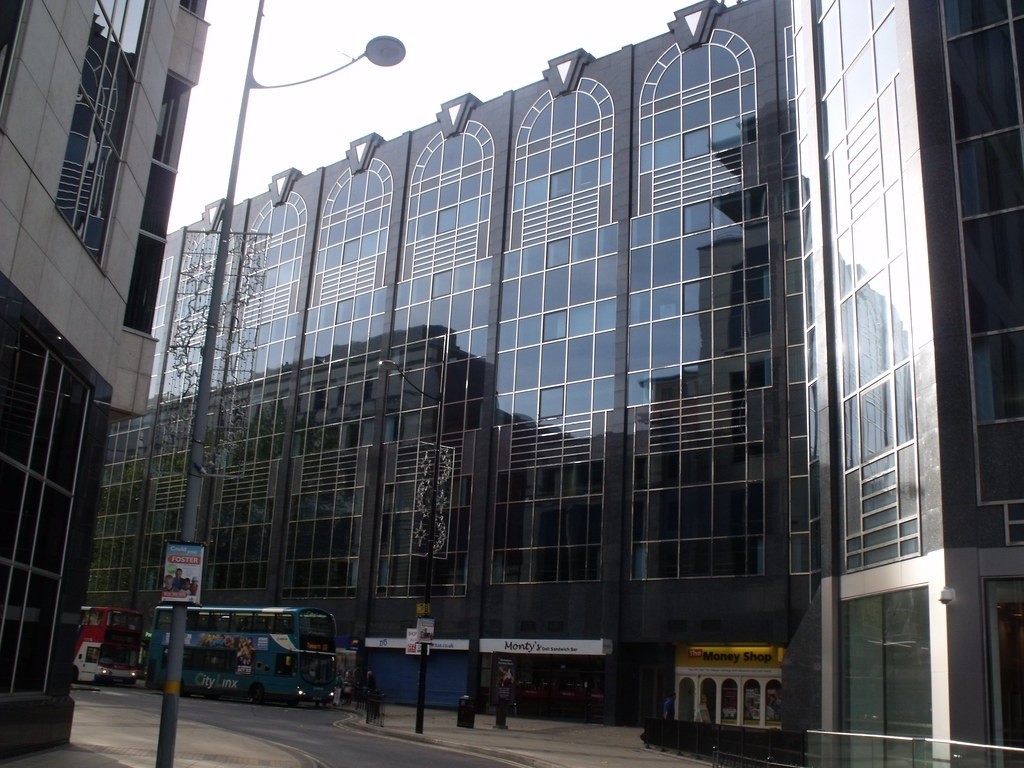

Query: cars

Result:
[513,668,607,718]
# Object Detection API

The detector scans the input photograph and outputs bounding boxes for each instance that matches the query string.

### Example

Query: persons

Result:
[163,568,199,595]
[663,693,677,721]
[333,670,376,708]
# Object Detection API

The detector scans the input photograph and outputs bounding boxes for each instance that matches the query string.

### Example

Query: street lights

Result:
[378,357,442,733]
[150,33,409,767]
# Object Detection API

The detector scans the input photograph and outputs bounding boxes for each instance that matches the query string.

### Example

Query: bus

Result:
[71,605,146,686]
[147,603,336,709]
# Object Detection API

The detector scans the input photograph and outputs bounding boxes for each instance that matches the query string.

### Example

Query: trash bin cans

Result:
[456,695,476,728]
